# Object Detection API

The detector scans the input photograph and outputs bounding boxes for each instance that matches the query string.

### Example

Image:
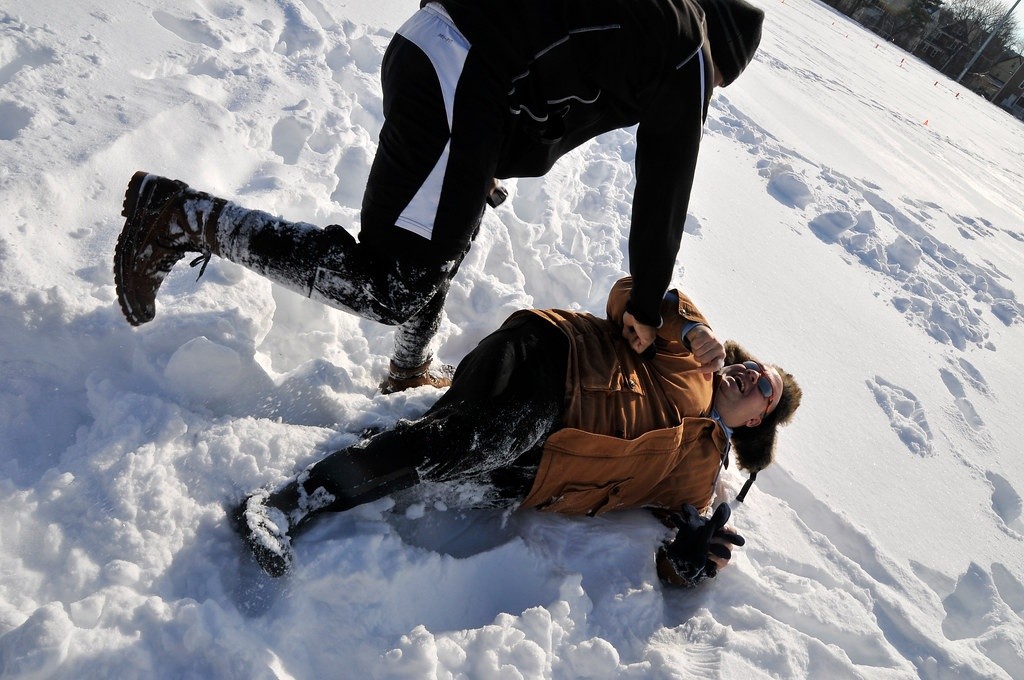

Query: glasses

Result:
[743,360,774,418]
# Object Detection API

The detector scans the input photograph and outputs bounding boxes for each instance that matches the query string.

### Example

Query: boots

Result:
[382,349,457,395]
[113,170,228,327]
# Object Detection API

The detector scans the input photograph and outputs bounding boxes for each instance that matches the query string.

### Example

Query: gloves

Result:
[656,501,746,589]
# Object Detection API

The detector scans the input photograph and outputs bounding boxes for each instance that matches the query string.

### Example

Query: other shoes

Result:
[229,487,293,576]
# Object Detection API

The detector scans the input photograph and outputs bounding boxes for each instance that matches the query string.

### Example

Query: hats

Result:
[698,0,764,87]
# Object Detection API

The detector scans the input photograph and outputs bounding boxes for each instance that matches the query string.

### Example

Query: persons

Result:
[112,1,765,395]
[230,277,801,591]
